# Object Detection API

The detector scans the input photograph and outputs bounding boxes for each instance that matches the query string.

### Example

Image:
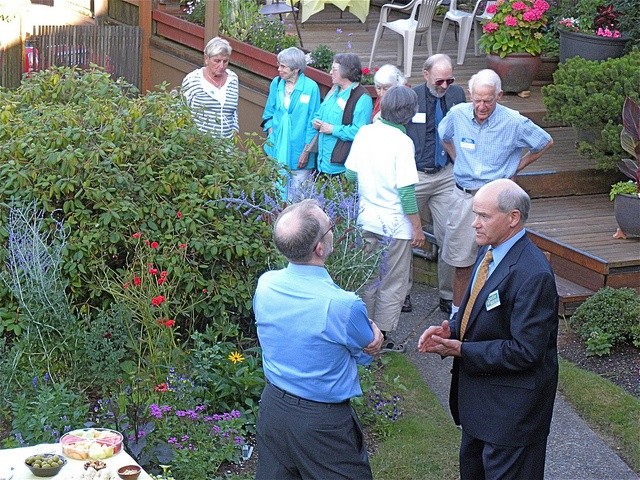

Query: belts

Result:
[456,182,477,196]
[417,157,451,174]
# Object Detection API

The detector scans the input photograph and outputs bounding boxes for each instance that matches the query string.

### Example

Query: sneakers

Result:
[401,295,412,312]
[439,298,453,316]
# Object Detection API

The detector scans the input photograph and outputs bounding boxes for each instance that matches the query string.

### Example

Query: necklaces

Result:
[204,68,227,90]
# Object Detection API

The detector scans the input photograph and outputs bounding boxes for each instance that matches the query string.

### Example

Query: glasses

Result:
[313,217,335,252]
[430,75,455,87]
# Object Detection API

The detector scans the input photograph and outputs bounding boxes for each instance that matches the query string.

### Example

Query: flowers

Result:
[558,3,622,39]
[476,0,551,60]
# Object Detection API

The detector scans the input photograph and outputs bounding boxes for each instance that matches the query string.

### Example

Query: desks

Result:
[286,0,370,25]
[1,443,153,480]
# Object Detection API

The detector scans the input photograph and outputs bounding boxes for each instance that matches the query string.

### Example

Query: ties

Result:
[460,251,494,343]
[435,98,448,169]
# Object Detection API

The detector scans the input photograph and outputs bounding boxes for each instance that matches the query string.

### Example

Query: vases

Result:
[557,27,631,65]
[485,50,541,92]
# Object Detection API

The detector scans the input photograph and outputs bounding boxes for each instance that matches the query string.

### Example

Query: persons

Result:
[251,198,387,479]
[369,64,407,124]
[416,177,560,480]
[343,84,427,355]
[400,52,467,319]
[258,45,321,202]
[178,35,240,155]
[308,51,374,190]
[436,67,555,327]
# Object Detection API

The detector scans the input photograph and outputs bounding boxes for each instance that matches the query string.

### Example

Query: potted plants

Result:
[610,96,640,240]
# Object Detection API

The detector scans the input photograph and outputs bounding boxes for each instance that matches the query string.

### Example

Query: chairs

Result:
[436,0,496,65]
[368,0,444,78]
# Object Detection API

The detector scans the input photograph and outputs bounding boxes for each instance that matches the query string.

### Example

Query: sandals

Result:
[379,336,405,353]
